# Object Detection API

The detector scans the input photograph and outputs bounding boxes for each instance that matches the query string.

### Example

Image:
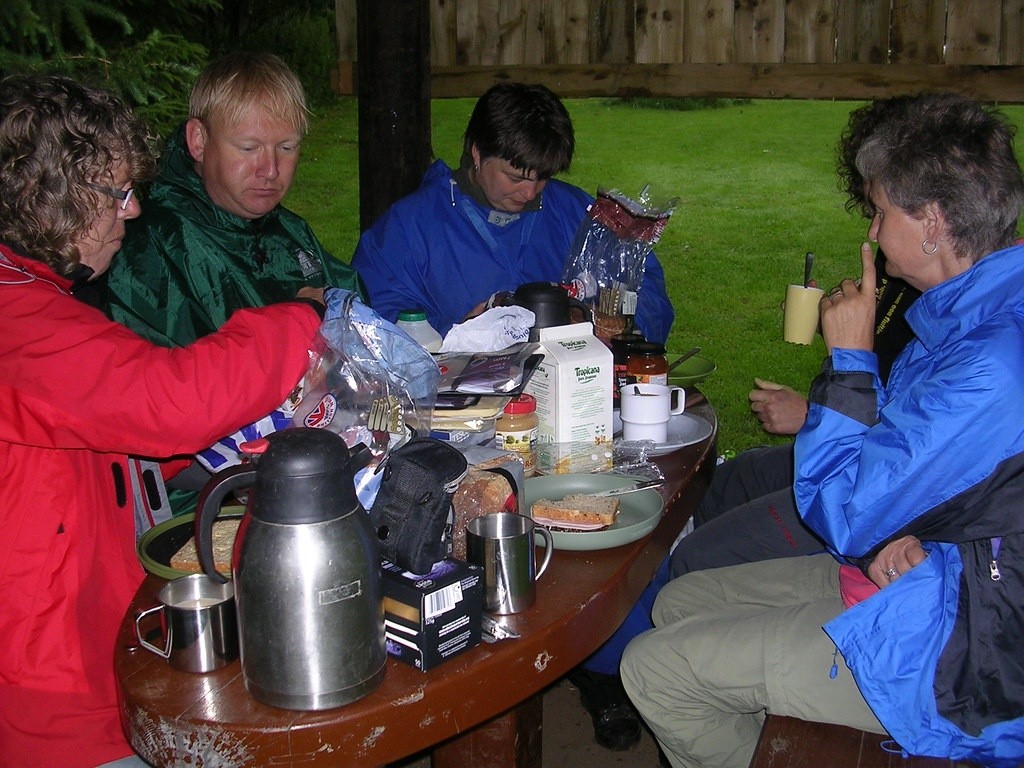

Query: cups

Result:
[783,285,825,345]
[619,383,686,442]
[131,574,240,673]
[464,511,553,615]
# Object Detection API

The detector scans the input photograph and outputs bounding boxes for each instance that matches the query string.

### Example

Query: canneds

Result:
[609,333,668,408]
[494,392,538,477]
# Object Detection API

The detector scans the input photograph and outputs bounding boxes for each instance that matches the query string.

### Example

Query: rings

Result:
[829,289,843,301]
[886,567,897,576]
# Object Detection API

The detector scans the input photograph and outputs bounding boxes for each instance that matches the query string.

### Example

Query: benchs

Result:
[748,714,984,768]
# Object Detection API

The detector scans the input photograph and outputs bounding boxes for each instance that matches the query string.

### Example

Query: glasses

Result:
[75,176,150,209]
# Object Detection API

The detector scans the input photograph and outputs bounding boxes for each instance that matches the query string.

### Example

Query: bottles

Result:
[393,308,443,354]
[610,333,648,406]
[627,343,669,387]
[496,393,539,477]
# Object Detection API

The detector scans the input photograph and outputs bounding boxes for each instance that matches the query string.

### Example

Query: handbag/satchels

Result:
[440,305,536,353]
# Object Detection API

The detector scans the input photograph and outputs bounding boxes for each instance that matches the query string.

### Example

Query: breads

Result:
[450,468,513,560]
[531,495,620,526]
[170,518,241,572]
[568,303,627,340]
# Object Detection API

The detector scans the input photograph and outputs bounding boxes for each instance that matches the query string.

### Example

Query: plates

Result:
[134,503,248,578]
[612,408,713,458]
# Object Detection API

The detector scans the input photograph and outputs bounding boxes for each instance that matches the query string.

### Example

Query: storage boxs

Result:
[372,557,484,674]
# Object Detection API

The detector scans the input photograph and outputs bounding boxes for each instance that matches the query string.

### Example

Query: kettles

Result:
[504,283,593,344]
[195,426,388,712]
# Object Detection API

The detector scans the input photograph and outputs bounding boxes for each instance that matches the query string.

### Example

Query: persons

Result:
[94,46,359,350]
[0,59,336,768]
[619,85,1023,768]
[666,94,925,578]
[352,81,674,753]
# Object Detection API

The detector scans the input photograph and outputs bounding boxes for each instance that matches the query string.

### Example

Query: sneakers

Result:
[578,669,642,753]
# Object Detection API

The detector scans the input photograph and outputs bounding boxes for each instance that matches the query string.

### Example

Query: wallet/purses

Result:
[370,438,468,576]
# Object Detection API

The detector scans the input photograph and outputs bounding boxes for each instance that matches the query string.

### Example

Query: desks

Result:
[114,385,718,768]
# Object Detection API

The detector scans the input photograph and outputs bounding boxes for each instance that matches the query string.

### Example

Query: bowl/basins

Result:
[520,474,664,550]
[666,352,718,391]
